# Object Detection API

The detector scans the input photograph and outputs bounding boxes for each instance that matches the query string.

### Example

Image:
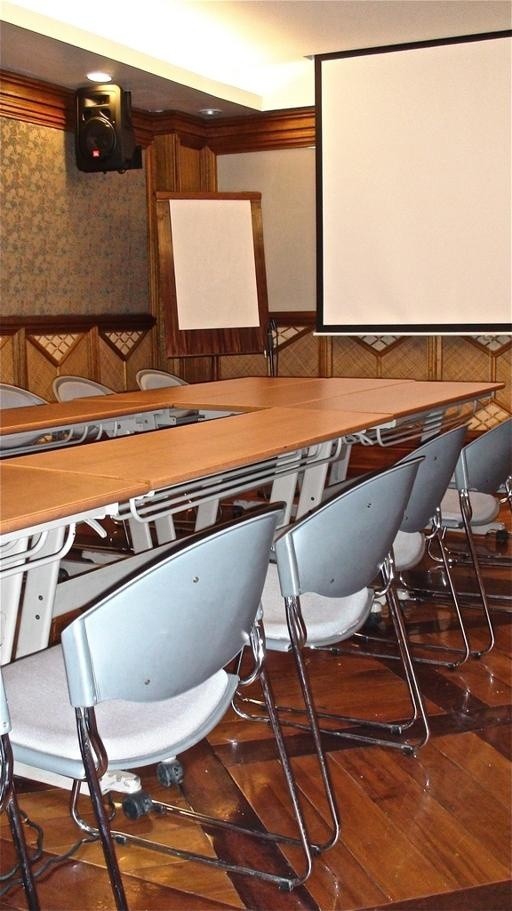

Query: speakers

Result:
[74,85,134,172]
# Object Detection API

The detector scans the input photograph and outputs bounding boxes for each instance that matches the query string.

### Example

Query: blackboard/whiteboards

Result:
[169,200,260,331]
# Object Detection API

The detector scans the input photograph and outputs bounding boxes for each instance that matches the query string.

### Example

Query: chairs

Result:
[2,368,196,457]
[350,413,510,658]
[117,455,431,854]
[2,500,292,910]
[228,418,466,738]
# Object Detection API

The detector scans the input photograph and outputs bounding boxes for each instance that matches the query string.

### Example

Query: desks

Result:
[2,460,144,666]
[5,408,396,593]
[0,399,180,551]
[171,376,416,524]
[289,378,505,483]
[112,375,302,541]
